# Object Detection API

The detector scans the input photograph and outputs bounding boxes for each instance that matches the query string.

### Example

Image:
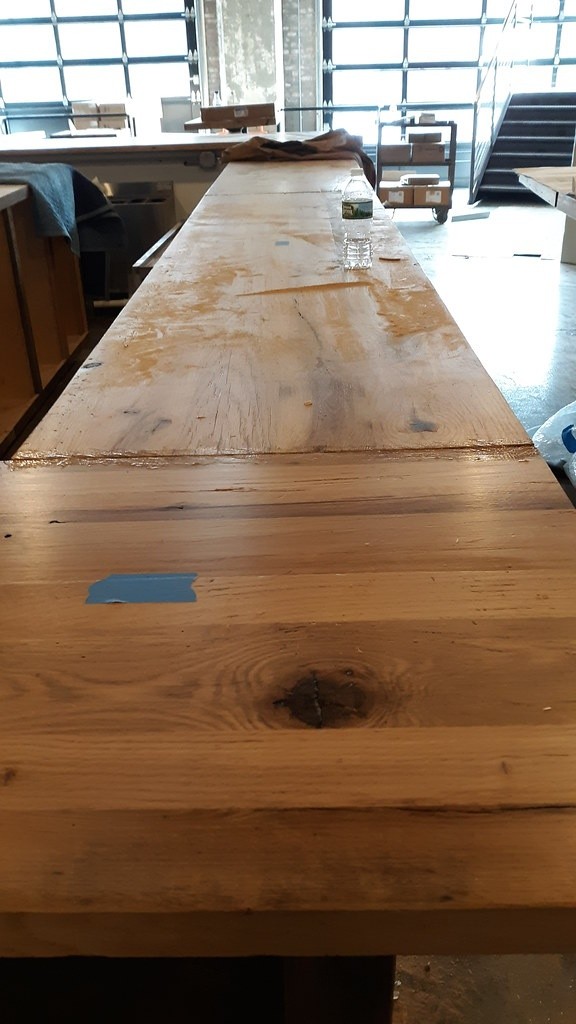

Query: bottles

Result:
[213,90,222,107]
[341,168,374,270]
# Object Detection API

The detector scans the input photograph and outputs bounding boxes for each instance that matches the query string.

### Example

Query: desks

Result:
[183,101,281,134]
[49,129,132,138]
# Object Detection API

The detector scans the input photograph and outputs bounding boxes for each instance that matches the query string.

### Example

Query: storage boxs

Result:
[419,113,437,125]
[406,173,441,186]
[412,143,445,166]
[71,101,127,130]
[200,102,275,122]
[379,144,411,166]
[409,132,442,143]
[378,180,413,207]
[413,180,451,208]
